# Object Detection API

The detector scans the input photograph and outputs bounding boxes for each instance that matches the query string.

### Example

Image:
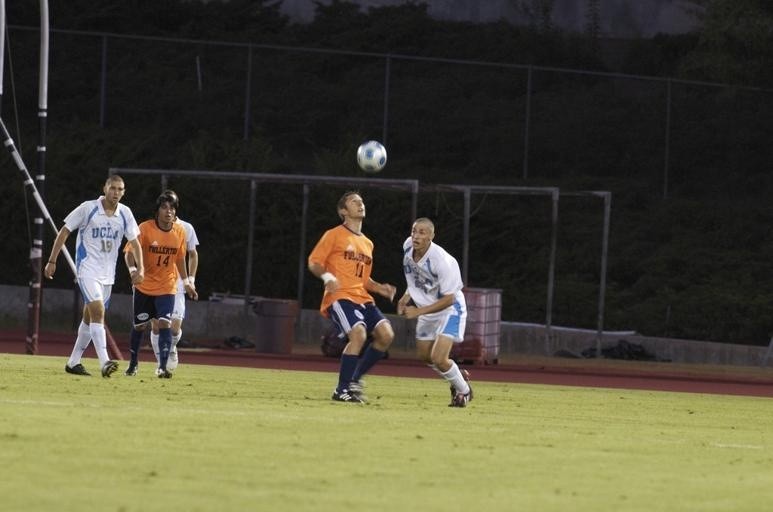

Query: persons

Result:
[396,218,475,410]
[309,191,397,405]
[150,190,199,371]
[44,174,145,378]
[124,194,200,378]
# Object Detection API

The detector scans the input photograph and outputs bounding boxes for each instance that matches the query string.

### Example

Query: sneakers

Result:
[350,381,368,401]
[332,387,363,403]
[157,367,172,378]
[168,346,178,363]
[65,362,90,375]
[449,369,471,398]
[124,361,139,376]
[447,385,473,407]
[101,360,119,377]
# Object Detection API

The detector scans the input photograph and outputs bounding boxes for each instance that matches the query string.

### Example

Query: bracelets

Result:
[48,261,56,264]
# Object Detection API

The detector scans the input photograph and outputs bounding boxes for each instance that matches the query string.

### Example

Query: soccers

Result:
[356,139,388,172]
